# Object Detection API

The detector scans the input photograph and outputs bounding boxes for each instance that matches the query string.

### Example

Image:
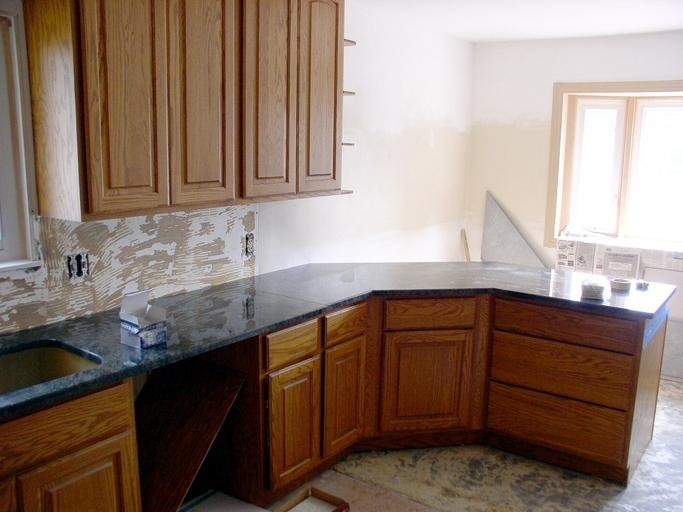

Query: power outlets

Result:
[245,232,254,255]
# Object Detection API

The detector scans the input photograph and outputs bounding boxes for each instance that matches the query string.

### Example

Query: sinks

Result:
[0,338,104,394]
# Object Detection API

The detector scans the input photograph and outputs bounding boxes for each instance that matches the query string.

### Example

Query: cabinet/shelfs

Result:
[22,0,355,223]
[0,295,670,512]
